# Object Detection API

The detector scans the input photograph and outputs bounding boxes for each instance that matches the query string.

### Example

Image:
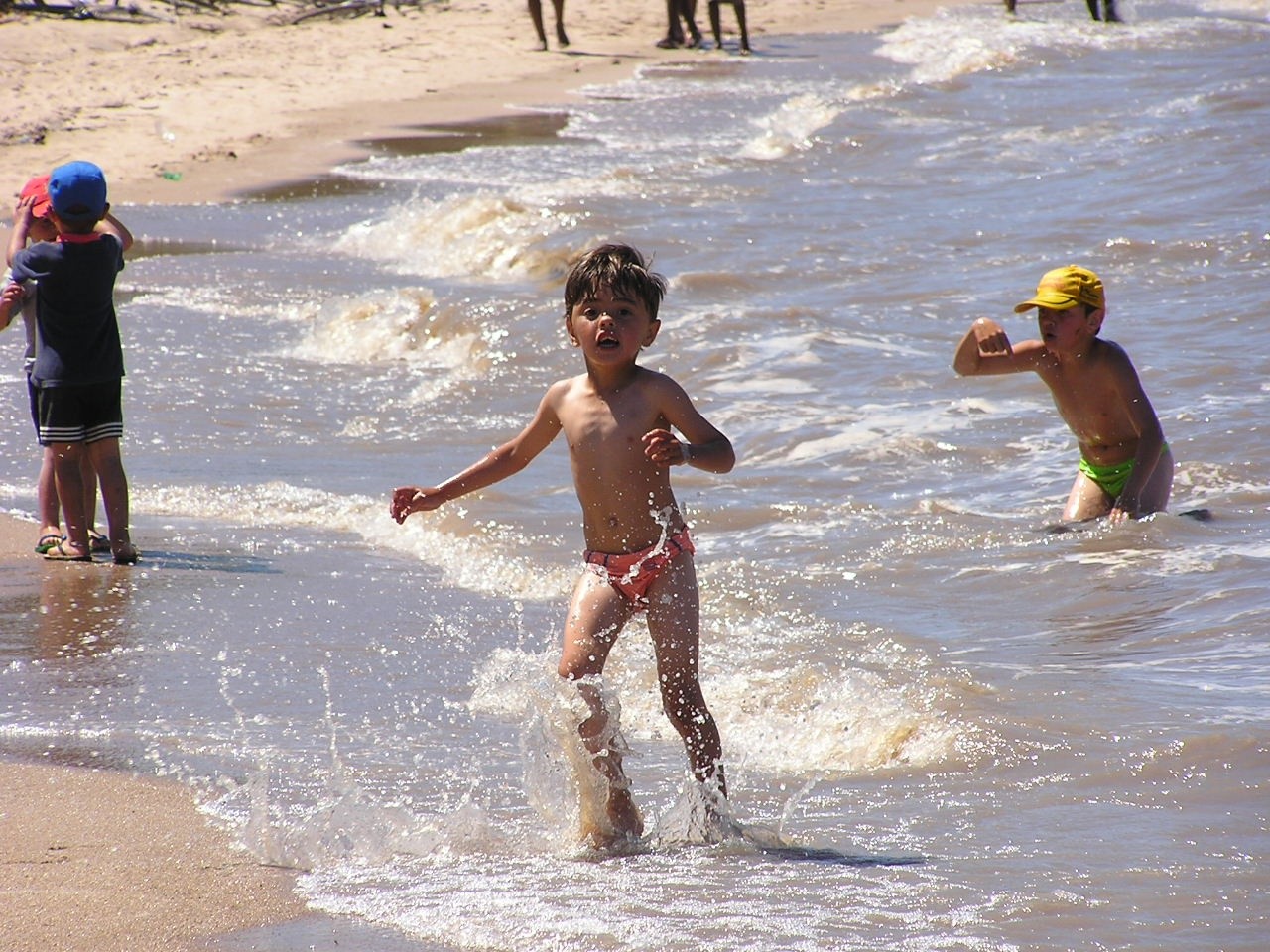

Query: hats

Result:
[19,174,54,217]
[1014,262,1106,314]
[47,160,107,220]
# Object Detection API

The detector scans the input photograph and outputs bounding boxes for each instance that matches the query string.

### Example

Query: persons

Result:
[1087,0,1123,22]
[390,244,744,849]
[0,160,141,565]
[1003,0,1016,11]
[951,264,1174,524]
[656,0,751,54]
[520,0,569,51]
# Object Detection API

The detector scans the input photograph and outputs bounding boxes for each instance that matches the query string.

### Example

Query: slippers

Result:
[88,533,110,551]
[43,545,92,562]
[35,534,67,552]
[113,546,139,564]
